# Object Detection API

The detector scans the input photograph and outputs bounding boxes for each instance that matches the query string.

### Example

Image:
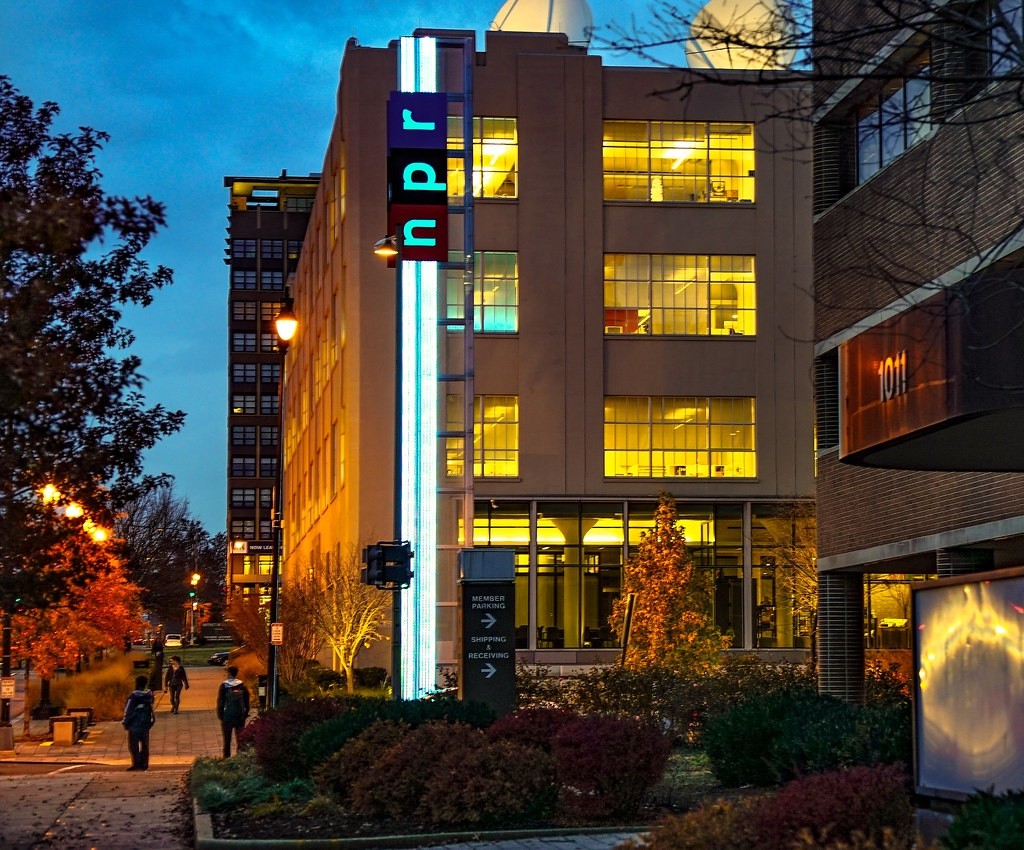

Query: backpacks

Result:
[224,688,245,724]
[128,702,151,734]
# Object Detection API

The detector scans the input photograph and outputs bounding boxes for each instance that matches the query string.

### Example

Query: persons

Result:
[164,656,189,714]
[216,666,250,757]
[760,596,771,604]
[151,638,164,656]
[121,676,155,772]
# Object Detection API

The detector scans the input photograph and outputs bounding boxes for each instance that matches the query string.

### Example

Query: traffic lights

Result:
[189,592,195,598]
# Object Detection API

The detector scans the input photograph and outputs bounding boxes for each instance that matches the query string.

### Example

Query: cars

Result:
[133,639,143,644]
[164,633,183,649]
[142,638,155,645]
[207,651,232,666]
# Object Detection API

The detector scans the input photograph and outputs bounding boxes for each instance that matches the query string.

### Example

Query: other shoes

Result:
[173,709,177,714]
[170,706,174,712]
[126,766,136,771]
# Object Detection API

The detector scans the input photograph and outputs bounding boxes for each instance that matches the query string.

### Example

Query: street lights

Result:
[372,233,408,689]
[262,286,300,714]
[188,573,200,644]
